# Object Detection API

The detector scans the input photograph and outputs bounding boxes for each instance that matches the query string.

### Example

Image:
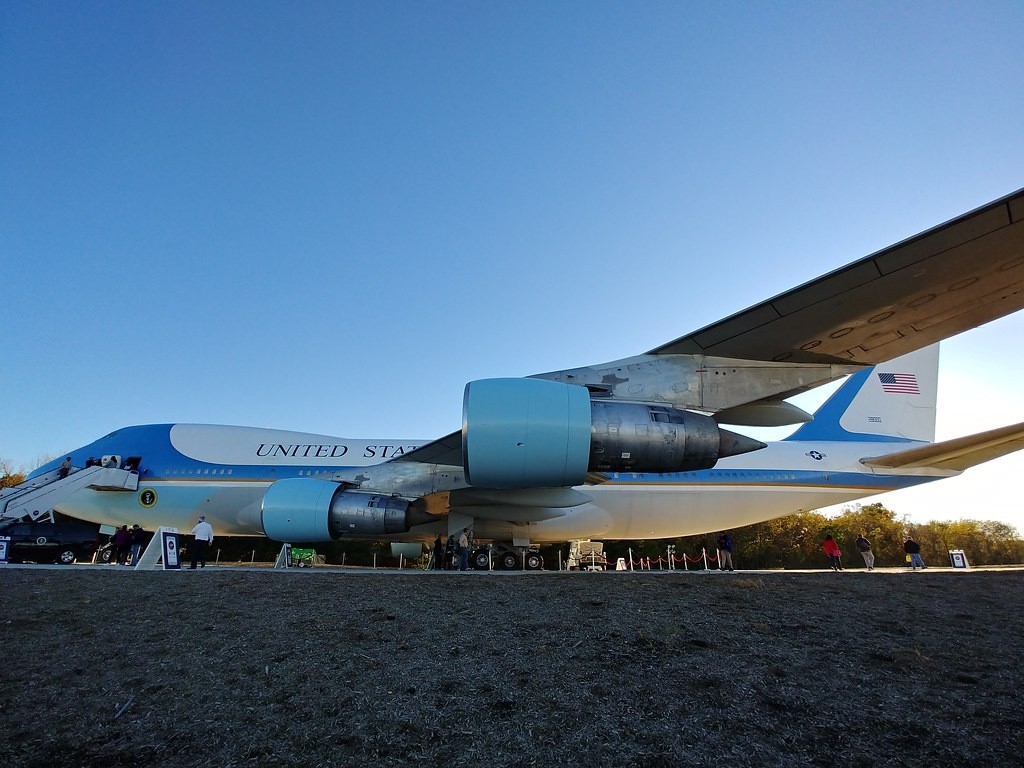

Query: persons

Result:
[855,533,874,571]
[187,516,214,569]
[433,534,444,571]
[58,457,72,481]
[823,535,844,570]
[904,536,927,570]
[86,456,135,471]
[106,524,145,566]
[717,532,734,571]
[442,528,479,570]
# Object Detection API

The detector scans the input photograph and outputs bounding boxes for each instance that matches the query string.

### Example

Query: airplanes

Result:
[0,189,1024,572]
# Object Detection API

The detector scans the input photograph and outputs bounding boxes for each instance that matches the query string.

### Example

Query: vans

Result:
[0,519,99,565]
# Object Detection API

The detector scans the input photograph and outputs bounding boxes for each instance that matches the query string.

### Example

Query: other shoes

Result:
[729,568,733,572]
[115,562,124,567]
[186,566,196,570]
[200,565,207,568]
[830,564,837,570]
[867,566,873,571]
[128,564,136,566]
[719,567,725,571]
[457,567,463,571]
[913,567,916,570]
[836,566,843,570]
[922,566,928,569]
[465,567,473,571]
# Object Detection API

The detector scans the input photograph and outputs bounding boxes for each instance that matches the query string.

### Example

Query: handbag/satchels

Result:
[726,533,733,553]
[832,549,842,557]
[905,553,912,562]
